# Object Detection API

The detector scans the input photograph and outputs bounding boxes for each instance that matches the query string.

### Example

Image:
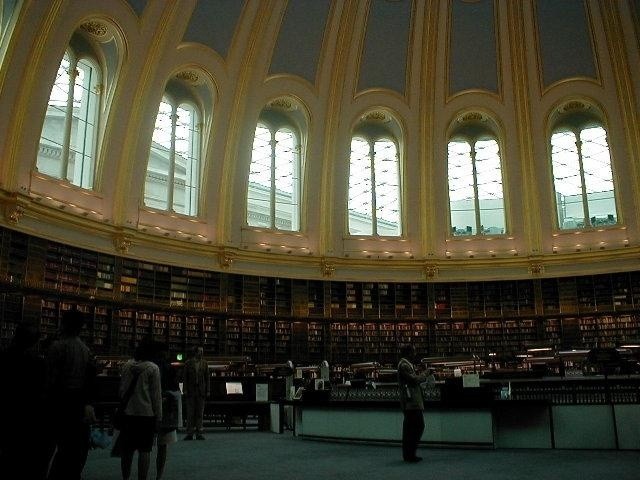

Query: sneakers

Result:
[182,433,205,441]
[405,456,423,463]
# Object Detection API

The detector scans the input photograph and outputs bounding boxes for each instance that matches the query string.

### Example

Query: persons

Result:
[397,342,437,463]
[0,307,212,480]
